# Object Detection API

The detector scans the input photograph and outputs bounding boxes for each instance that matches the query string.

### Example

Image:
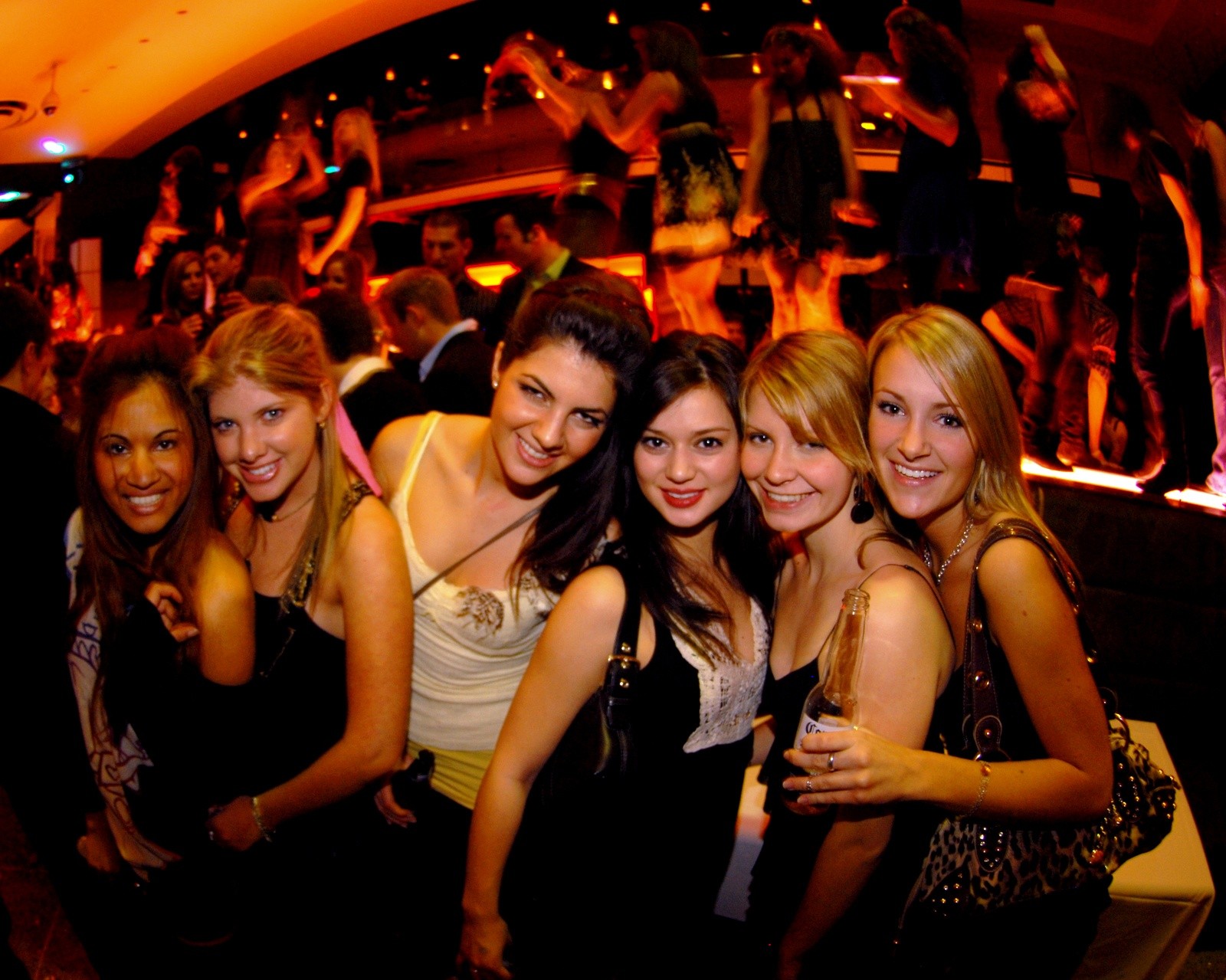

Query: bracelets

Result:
[251,794,278,842]
[966,758,992,817]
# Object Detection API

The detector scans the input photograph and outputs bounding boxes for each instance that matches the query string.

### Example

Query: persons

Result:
[51,332,288,979]
[456,328,771,979]
[0,0,1226,980]
[785,305,1115,979]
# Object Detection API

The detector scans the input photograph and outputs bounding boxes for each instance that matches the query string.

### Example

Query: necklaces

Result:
[922,512,977,588]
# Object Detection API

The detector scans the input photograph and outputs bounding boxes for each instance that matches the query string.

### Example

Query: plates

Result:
[840,74,902,85]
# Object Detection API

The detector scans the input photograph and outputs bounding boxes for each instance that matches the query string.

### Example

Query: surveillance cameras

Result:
[41,91,61,116]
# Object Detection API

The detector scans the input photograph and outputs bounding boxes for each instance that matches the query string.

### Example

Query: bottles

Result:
[370,749,435,865]
[783,588,871,815]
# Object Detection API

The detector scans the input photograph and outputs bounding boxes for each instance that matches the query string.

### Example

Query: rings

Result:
[826,751,837,771]
[207,829,216,842]
[806,775,815,794]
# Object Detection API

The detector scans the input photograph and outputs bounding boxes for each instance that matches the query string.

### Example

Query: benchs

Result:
[1098,720,1215,980]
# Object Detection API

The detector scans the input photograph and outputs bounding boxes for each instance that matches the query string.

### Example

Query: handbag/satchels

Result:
[501,554,642,883]
[893,727,1182,946]
